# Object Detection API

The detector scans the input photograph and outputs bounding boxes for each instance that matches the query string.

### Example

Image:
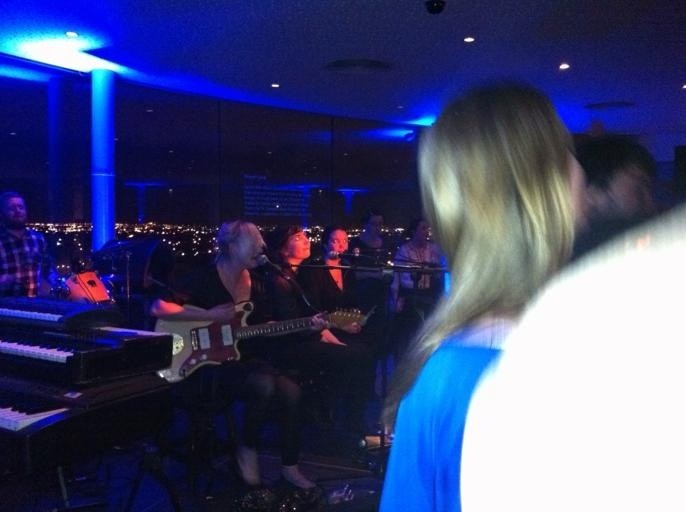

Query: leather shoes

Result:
[280,464,315,489]
[235,446,260,487]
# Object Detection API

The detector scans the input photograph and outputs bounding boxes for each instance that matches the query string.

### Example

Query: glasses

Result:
[328,240,351,245]
[288,233,309,240]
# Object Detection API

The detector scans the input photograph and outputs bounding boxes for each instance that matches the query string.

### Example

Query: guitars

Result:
[152,299,366,388]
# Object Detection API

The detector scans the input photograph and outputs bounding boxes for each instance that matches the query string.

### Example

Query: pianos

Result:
[2,293,122,330]
[2,371,176,444]
[1,325,175,384]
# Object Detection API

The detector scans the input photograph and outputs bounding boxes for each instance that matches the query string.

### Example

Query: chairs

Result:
[184,381,241,460]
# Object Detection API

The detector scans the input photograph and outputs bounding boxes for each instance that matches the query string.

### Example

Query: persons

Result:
[150,220,313,489]
[318,226,352,335]
[378,81,582,512]
[0,191,70,300]
[393,222,444,313]
[271,219,345,425]
[350,207,398,308]
[571,130,655,258]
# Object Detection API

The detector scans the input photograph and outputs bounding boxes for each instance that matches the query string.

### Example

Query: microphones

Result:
[256,255,281,273]
[329,253,352,258]
